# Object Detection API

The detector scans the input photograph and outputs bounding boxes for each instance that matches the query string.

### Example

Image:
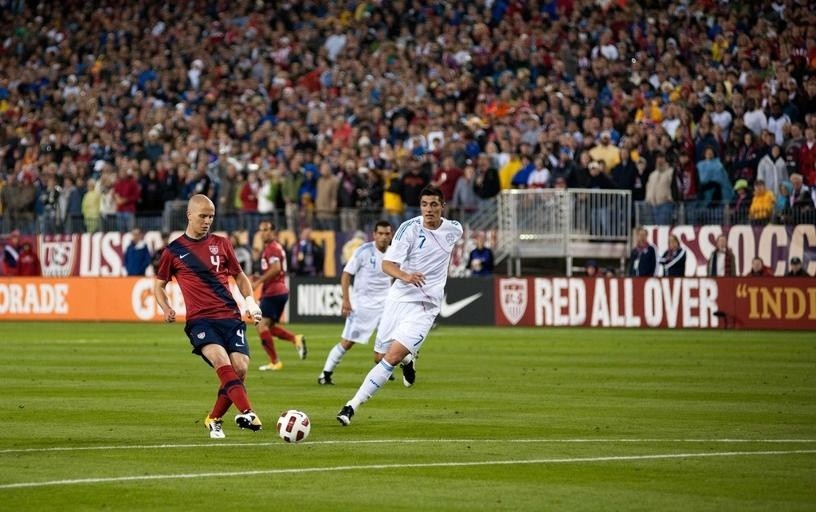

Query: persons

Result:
[0,4,816,279]
[252,218,307,372]
[152,194,263,439]
[317,222,394,386]
[338,188,464,427]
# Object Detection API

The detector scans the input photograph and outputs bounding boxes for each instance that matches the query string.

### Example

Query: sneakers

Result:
[235,408,263,433]
[400,354,416,388]
[375,360,396,382]
[204,412,226,440]
[318,370,335,386]
[293,333,308,361]
[335,404,356,427]
[257,359,284,372]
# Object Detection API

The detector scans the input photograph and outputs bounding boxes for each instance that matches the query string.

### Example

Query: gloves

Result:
[242,294,263,324]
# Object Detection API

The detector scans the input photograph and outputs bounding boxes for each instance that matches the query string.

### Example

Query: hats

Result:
[734,178,749,191]
[790,256,800,265]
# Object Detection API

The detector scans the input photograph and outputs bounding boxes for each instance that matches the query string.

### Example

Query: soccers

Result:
[277,410,309,442]
